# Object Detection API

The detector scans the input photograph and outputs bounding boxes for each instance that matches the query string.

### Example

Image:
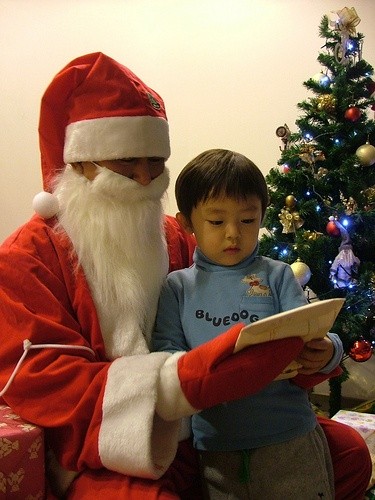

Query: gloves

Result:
[155,322,305,423]
[288,365,343,391]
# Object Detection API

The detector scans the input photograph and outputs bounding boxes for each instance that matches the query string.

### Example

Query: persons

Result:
[153,149,344,500]
[0,51,372,500]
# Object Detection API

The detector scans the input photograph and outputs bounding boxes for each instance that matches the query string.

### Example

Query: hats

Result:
[32,52,171,218]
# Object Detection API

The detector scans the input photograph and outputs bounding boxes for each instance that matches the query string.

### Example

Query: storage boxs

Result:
[330,410,375,491]
